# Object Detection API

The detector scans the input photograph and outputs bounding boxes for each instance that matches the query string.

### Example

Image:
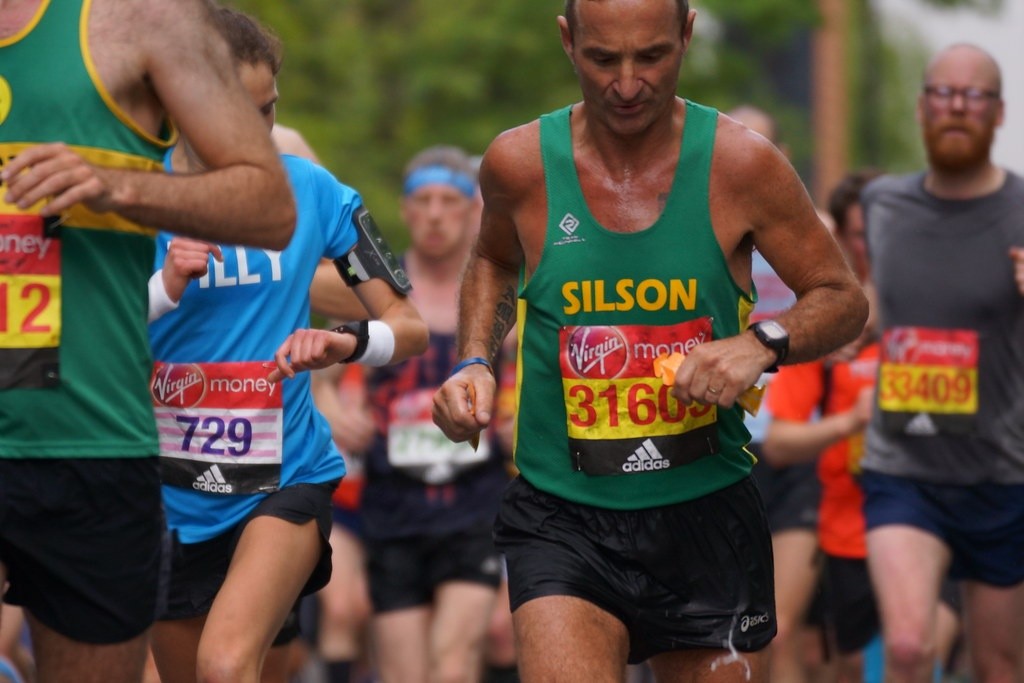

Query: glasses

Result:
[924,86,999,102]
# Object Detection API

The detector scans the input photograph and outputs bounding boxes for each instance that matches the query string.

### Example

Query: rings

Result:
[707,387,721,394]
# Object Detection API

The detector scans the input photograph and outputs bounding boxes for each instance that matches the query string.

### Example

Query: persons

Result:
[260,123,519,683]
[433,0,869,683]
[151,8,429,683]
[0,0,298,683]
[718,45,1024,683]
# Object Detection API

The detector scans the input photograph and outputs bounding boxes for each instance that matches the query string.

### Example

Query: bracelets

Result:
[451,358,492,377]
[147,270,178,321]
[358,321,395,367]
[332,320,369,365]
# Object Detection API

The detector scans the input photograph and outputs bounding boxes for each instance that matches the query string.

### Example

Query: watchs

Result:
[747,319,790,373]
